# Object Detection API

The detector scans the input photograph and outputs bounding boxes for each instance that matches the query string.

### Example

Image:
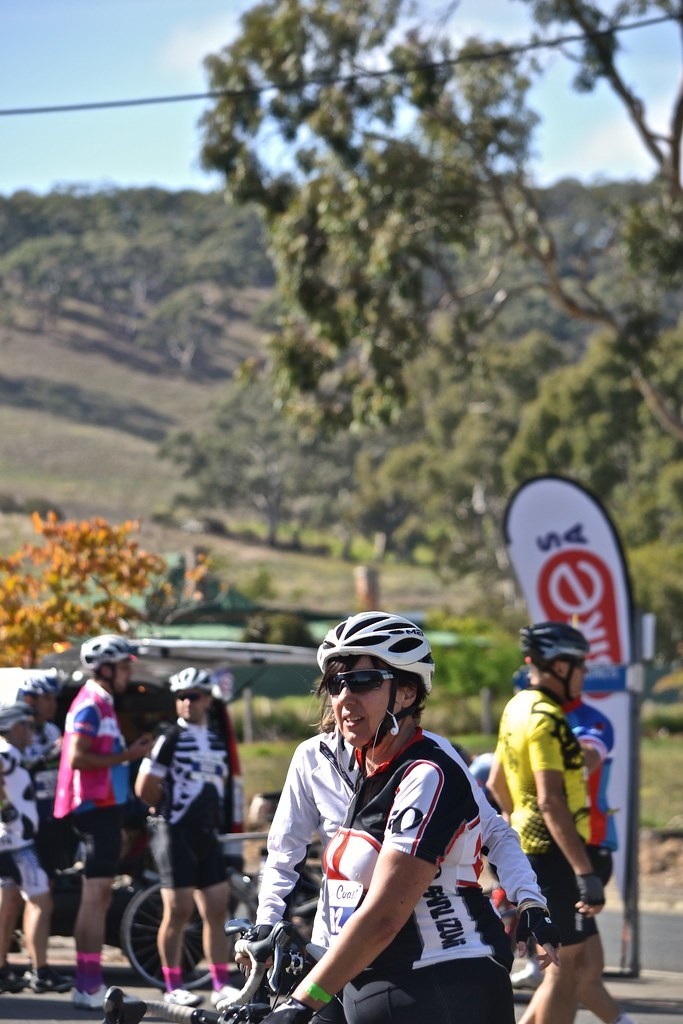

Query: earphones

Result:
[390,717,399,735]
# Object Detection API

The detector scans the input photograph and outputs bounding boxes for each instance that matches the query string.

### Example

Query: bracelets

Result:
[300,976,332,1003]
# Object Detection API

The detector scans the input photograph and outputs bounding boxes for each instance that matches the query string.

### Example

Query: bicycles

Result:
[101,915,374,1024]
[119,806,326,992]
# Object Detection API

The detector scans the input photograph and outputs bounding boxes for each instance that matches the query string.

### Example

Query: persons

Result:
[136,666,242,1005]
[254,708,561,1024]
[234,611,519,1023]
[0,666,79,996]
[514,662,636,1023]
[454,741,504,819]
[487,618,606,1024]
[53,634,156,1009]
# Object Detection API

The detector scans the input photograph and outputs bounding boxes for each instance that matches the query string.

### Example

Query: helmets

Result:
[80,635,130,669]
[512,664,532,689]
[19,675,63,697]
[518,621,590,671]
[0,702,34,732]
[168,667,214,693]
[317,611,436,694]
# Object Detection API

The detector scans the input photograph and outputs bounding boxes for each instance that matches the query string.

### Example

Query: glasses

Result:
[177,690,210,701]
[325,668,396,695]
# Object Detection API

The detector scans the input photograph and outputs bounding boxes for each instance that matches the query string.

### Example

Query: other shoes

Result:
[163,986,205,1007]
[509,965,544,990]
[210,986,248,1007]
[76,984,139,1009]
[0,971,26,995]
[29,972,72,995]
